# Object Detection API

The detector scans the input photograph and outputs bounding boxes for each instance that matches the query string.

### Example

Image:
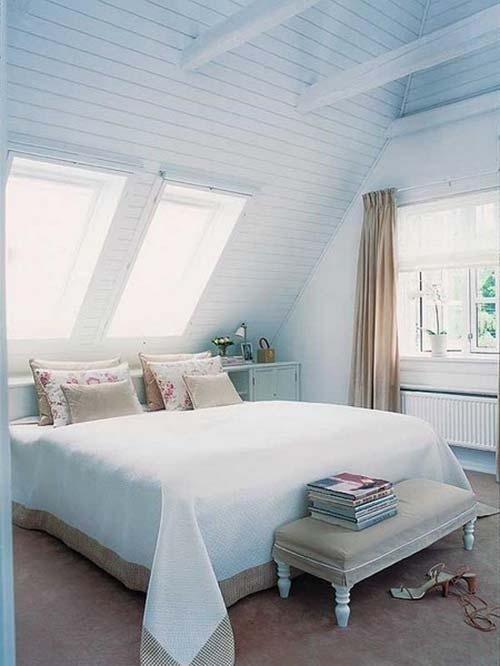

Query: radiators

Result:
[400,391,497,454]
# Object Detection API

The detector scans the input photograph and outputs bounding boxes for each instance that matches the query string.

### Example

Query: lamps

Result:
[235,323,251,363]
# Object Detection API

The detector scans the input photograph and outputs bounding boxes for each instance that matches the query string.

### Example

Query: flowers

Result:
[211,336,234,356]
[425,283,448,335]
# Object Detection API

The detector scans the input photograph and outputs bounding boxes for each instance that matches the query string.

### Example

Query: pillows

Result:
[60,379,140,423]
[181,371,242,409]
[138,351,211,412]
[146,356,224,412]
[34,362,142,426]
[29,356,123,427]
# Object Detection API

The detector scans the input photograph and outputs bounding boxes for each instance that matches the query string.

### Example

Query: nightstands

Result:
[223,362,302,402]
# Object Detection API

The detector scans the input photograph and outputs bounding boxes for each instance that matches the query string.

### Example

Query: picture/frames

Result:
[240,342,254,362]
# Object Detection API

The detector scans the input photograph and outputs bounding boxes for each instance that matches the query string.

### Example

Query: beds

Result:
[7,376,479,665]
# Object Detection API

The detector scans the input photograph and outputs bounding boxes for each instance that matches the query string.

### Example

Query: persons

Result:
[244,345,253,359]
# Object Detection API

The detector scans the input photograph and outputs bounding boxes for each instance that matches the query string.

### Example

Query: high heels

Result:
[389,569,454,600]
[424,562,478,594]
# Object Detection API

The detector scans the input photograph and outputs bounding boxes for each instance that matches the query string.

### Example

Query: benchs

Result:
[269,477,478,627]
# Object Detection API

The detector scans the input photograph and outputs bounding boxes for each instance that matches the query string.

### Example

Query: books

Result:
[306,471,400,532]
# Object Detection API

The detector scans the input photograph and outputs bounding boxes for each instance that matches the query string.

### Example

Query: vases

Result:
[428,333,448,354]
